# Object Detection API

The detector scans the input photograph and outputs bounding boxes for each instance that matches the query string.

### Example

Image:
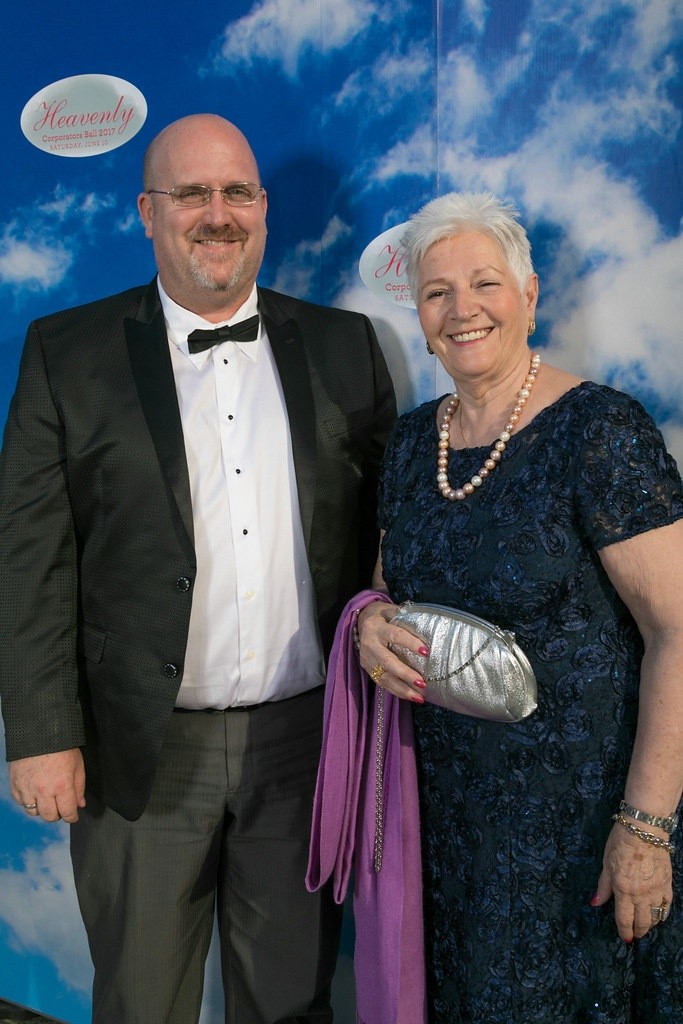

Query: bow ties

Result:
[187,314,261,354]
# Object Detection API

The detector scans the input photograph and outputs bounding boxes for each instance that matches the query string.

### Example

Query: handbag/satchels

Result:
[389,602,539,725]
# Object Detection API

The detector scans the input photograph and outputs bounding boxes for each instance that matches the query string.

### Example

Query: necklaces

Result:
[438,351,541,502]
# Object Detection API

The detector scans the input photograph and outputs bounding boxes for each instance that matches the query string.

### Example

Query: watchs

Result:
[619,800,678,834]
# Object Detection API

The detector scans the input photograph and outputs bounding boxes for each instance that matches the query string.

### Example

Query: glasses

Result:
[148,181,263,209]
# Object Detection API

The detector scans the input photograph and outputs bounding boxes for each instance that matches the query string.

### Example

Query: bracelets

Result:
[351,610,361,655]
[614,815,675,856]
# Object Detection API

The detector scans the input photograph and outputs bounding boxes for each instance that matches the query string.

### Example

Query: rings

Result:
[22,804,39,809]
[369,663,385,683]
[651,900,671,920]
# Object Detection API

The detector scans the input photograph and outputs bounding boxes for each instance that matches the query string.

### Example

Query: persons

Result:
[0,107,401,1024]
[353,190,683,1023]
